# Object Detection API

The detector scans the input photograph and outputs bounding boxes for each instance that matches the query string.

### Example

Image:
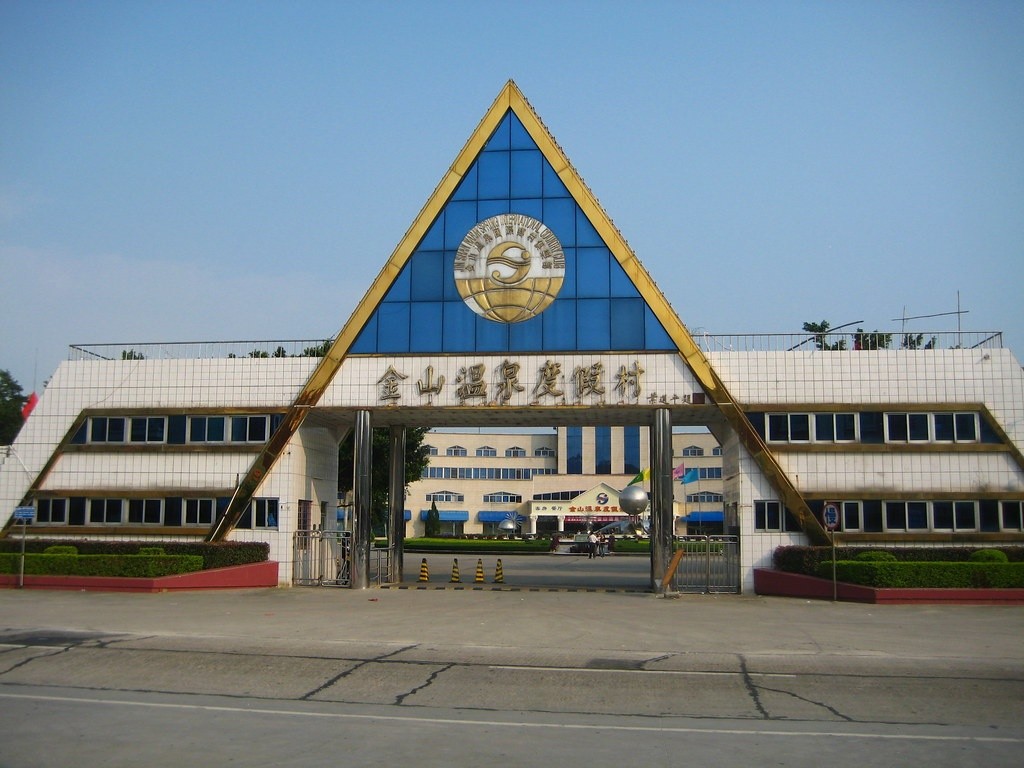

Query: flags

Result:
[673,462,684,480]
[681,469,699,485]
[627,469,644,486]
[643,467,650,480]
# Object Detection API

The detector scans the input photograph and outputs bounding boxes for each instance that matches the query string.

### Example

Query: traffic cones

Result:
[472,558,486,584]
[417,558,432,582]
[448,558,462,583]
[491,558,506,584]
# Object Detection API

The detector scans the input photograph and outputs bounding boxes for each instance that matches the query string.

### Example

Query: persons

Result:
[551,529,615,559]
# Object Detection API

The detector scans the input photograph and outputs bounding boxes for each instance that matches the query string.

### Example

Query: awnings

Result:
[421,510,469,521]
[404,510,411,520]
[680,511,723,522]
[478,511,526,522]
